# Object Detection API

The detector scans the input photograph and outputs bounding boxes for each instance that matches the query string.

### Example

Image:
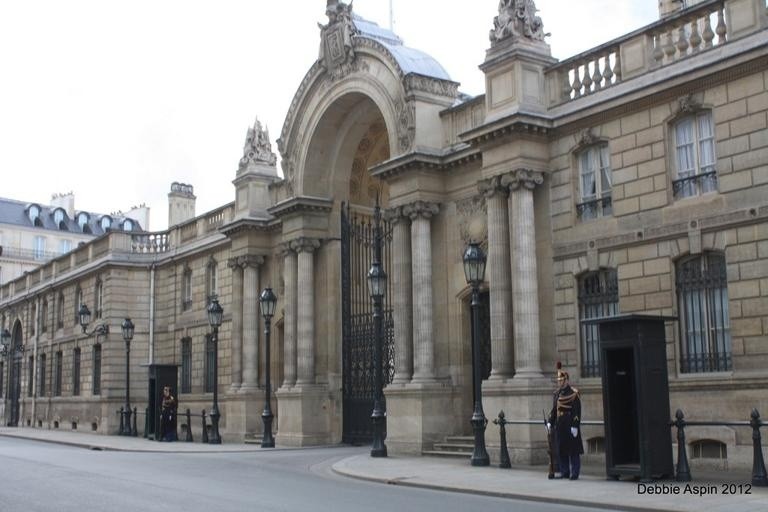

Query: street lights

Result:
[366,261,389,456]
[207,299,224,444]
[462,239,490,465]
[260,286,277,449]
[121,317,135,433]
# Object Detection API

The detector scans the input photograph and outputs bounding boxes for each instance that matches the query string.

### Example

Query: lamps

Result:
[79,305,108,339]
[1,330,25,355]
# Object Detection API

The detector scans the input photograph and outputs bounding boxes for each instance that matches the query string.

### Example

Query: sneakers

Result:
[552,474,578,480]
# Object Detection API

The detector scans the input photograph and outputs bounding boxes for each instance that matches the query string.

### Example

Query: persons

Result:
[546,369,584,480]
[159,385,176,441]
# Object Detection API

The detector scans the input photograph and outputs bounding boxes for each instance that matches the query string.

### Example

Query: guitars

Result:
[543,410,555,479]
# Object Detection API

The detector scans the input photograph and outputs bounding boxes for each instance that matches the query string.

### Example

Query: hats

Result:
[163,383,172,390]
[555,369,570,381]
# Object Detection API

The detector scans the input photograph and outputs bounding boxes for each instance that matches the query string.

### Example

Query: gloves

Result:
[569,427,578,438]
[544,423,551,433]
